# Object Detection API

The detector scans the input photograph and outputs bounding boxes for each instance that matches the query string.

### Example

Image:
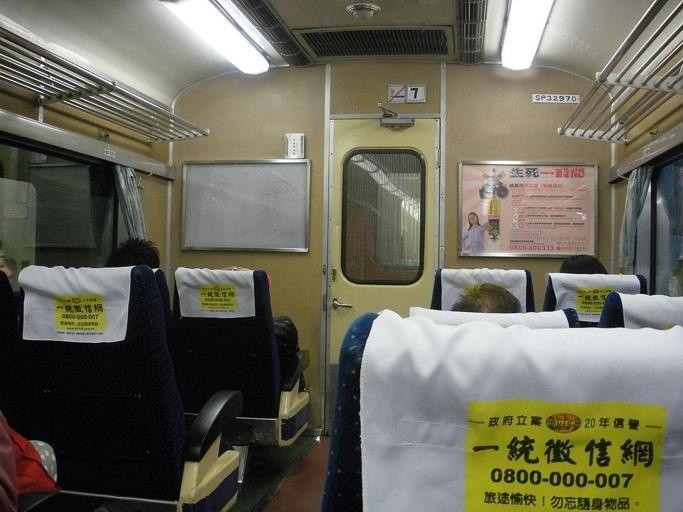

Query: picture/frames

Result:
[455,158,600,259]
[179,159,312,254]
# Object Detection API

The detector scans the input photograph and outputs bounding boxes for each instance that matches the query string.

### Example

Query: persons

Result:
[560,253,606,274]
[105,234,204,414]
[224,259,305,392]
[461,213,488,254]
[449,282,525,311]
[0,240,25,319]
[0,405,58,511]
